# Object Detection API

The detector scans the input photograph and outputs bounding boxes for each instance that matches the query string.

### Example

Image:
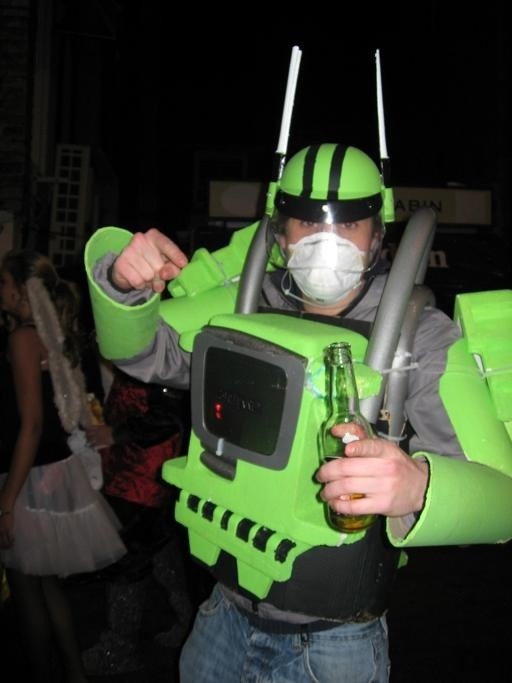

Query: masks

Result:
[283,231,381,303]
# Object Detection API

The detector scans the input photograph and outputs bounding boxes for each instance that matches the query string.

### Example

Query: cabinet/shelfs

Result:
[47,140,101,254]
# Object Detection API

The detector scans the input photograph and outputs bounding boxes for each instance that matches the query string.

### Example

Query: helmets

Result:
[274,143,383,223]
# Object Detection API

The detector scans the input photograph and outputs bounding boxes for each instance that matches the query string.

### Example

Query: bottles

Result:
[318,343,379,533]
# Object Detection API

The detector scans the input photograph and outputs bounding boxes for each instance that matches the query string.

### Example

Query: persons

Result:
[0,249,126,683]
[80,359,196,676]
[83,143,512,683]
